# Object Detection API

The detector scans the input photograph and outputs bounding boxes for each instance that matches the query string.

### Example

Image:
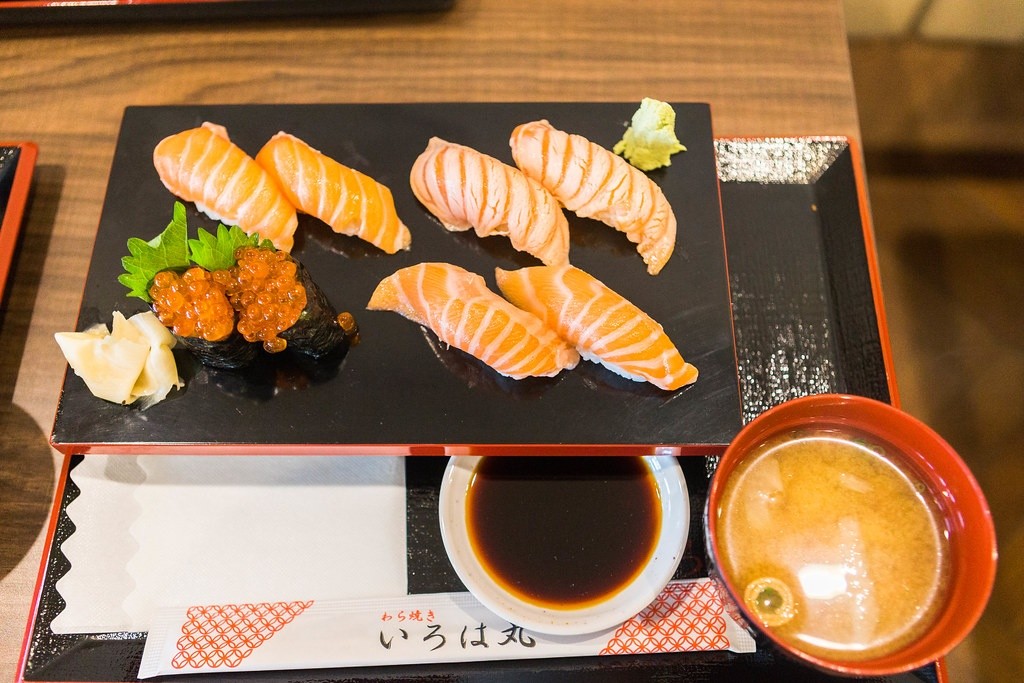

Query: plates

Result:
[53,97,744,455]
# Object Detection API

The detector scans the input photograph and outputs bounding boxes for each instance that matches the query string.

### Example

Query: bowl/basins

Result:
[438,452,691,635]
[704,395,998,679]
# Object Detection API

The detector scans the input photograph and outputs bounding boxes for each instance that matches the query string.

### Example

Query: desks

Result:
[2,0,950,682]
